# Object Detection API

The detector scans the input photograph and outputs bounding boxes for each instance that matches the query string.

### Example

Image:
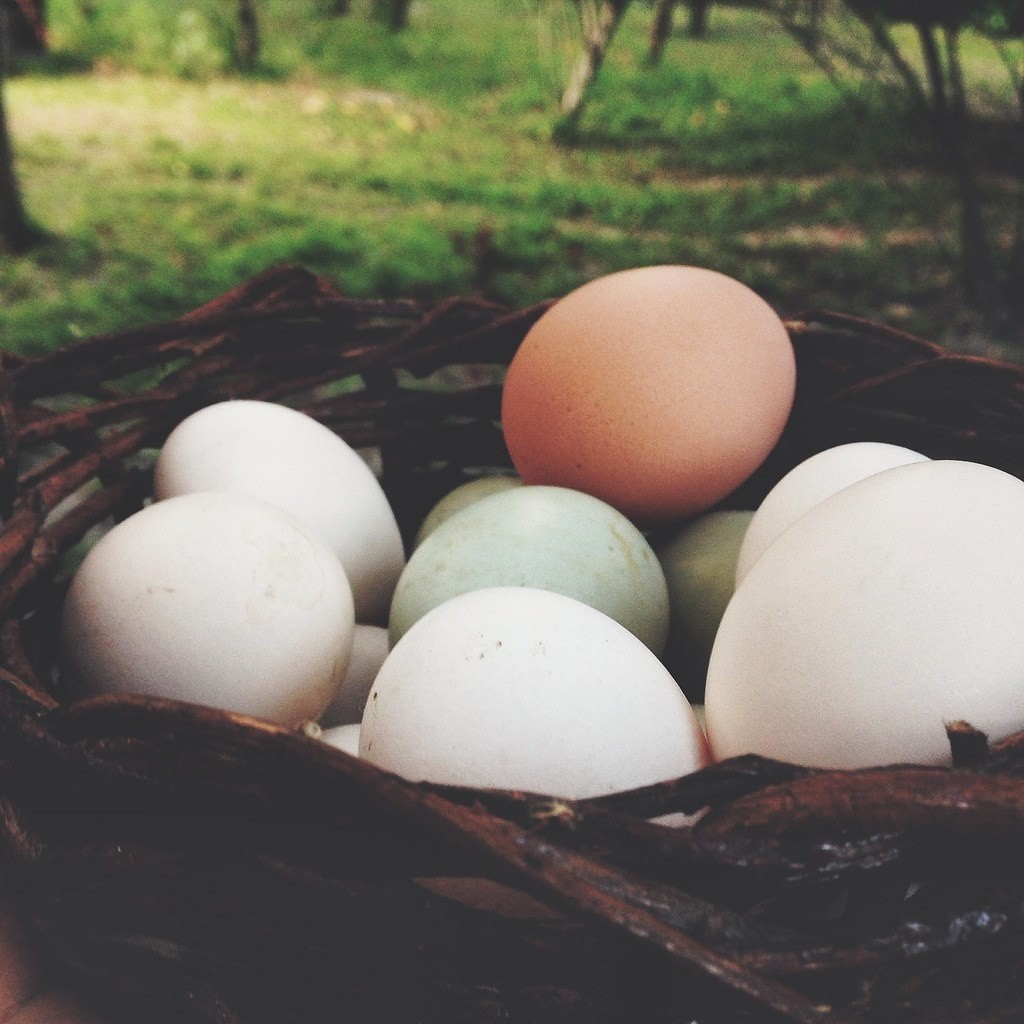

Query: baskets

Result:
[2,258,1023,1022]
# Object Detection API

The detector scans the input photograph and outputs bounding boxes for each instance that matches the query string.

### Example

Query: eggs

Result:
[51,263,1023,926]
